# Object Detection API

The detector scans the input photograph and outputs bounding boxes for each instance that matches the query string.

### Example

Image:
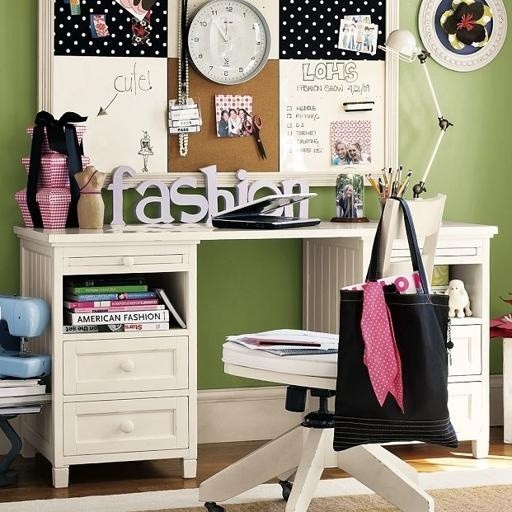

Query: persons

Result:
[219,109,252,135]
[332,138,372,164]
[339,184,358,218]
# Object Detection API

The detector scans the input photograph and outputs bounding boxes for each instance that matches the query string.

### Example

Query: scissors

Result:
[243,115,266,160]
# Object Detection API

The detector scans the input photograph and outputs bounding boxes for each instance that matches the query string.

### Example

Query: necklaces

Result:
[179,0,190,157]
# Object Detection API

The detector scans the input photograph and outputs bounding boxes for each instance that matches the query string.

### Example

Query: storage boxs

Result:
[14,187,73,228]
[21,152,89,188]
[27,127,85,153]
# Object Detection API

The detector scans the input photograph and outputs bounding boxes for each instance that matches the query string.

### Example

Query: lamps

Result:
[378,29,448,221]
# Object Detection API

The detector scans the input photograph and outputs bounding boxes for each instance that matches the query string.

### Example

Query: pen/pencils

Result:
[388,168,392,185]
[402,182,409,194]
[369,173,380,193]
[382,167,388,184]
[398,164,403,189]
[378,177,383,196]
[396,173,411,196]
[388,173,392,198]
[365,174,373,185]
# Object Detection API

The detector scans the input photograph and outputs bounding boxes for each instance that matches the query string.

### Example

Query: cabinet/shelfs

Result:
[303,222,499,458]
[14,225,198,487]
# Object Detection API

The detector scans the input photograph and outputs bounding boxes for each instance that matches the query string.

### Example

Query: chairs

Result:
[199,193,447,511]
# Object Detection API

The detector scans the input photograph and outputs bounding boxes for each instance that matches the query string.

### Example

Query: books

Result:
[0,379,53,416]
[63,285,186,332]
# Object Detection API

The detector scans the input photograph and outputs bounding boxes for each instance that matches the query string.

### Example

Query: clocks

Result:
[186,1,271,86]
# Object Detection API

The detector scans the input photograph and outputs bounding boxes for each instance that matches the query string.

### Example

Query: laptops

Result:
[212,191,321,230]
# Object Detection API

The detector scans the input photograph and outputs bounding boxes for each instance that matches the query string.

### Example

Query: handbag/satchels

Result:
[332,195,460,452]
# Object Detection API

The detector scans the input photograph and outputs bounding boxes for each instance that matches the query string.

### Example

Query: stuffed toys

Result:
[445,278,473,318]
